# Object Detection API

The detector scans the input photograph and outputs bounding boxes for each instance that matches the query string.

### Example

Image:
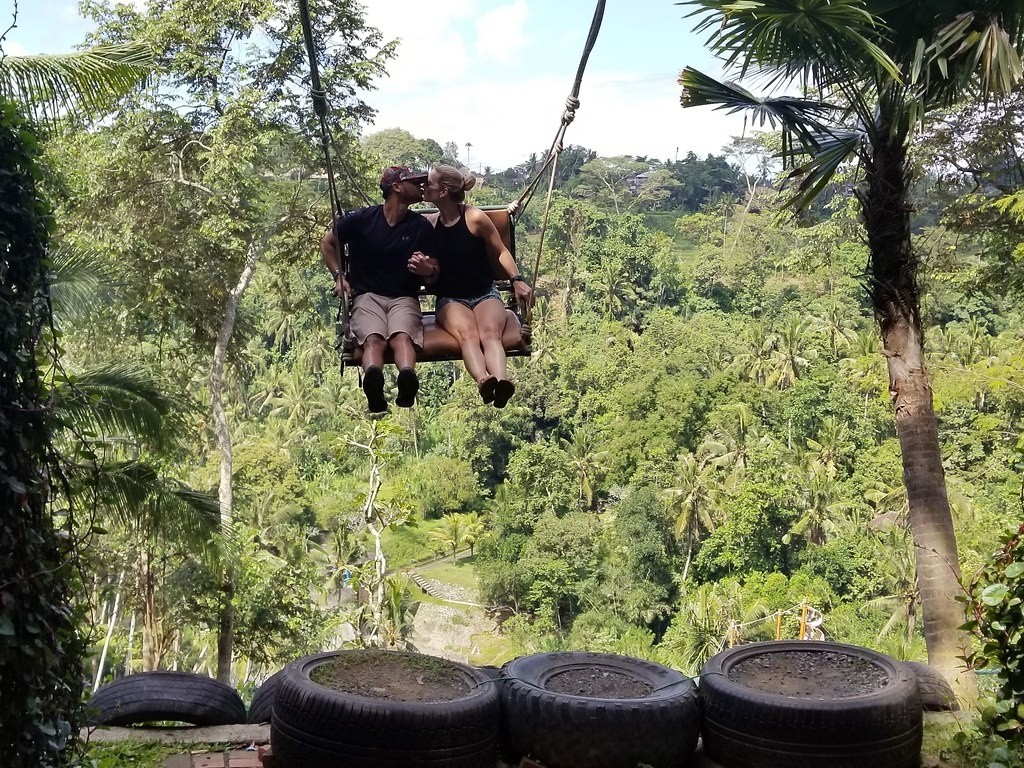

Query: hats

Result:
[380,165,427,188]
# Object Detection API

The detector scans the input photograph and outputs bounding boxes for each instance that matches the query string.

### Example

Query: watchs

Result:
[510,274,525,286]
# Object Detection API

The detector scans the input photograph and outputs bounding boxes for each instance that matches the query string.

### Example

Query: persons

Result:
[319,165,441,413]
[409,165,538,409]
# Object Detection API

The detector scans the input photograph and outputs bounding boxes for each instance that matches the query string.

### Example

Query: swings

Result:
[295,0,606,387]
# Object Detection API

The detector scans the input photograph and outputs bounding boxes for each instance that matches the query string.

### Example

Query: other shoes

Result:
[363,366,387,412]
[395,367,419,408]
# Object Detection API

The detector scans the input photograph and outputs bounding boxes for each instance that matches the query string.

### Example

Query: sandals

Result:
[478,375,497,404]
[494,380,515,408]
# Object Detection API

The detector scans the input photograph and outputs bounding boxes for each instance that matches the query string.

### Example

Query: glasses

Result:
[404,179,420,185]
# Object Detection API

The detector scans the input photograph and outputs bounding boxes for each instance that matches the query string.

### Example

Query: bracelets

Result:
[332,268,344,281]
[426,265,437,278]
